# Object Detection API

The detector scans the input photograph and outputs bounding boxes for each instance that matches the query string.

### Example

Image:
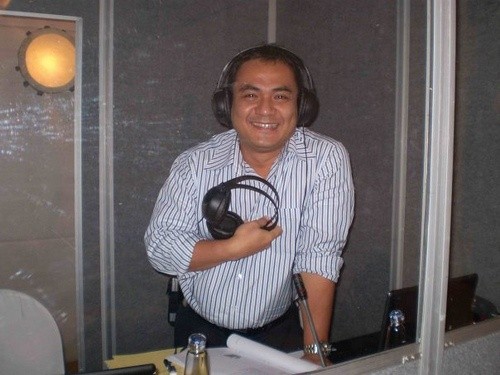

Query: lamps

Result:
[16,25,78,95]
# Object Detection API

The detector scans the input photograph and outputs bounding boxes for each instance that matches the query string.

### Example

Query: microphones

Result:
[291,273,325,369]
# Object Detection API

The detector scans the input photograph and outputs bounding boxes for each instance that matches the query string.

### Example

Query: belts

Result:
[180,300,296,339]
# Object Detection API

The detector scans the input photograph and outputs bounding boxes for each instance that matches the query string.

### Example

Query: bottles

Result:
[384,309,406,351]
[183,333,209,375]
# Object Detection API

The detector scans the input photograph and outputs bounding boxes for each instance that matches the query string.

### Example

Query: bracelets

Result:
[303,341,332,357]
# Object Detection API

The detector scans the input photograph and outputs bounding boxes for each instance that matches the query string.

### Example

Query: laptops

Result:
[376,274,478,352]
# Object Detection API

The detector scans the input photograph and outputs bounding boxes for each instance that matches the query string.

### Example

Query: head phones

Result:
[212,44,319,129]
[202,175,280,240]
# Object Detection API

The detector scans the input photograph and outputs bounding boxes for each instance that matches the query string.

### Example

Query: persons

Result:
[144,43,355,368]
